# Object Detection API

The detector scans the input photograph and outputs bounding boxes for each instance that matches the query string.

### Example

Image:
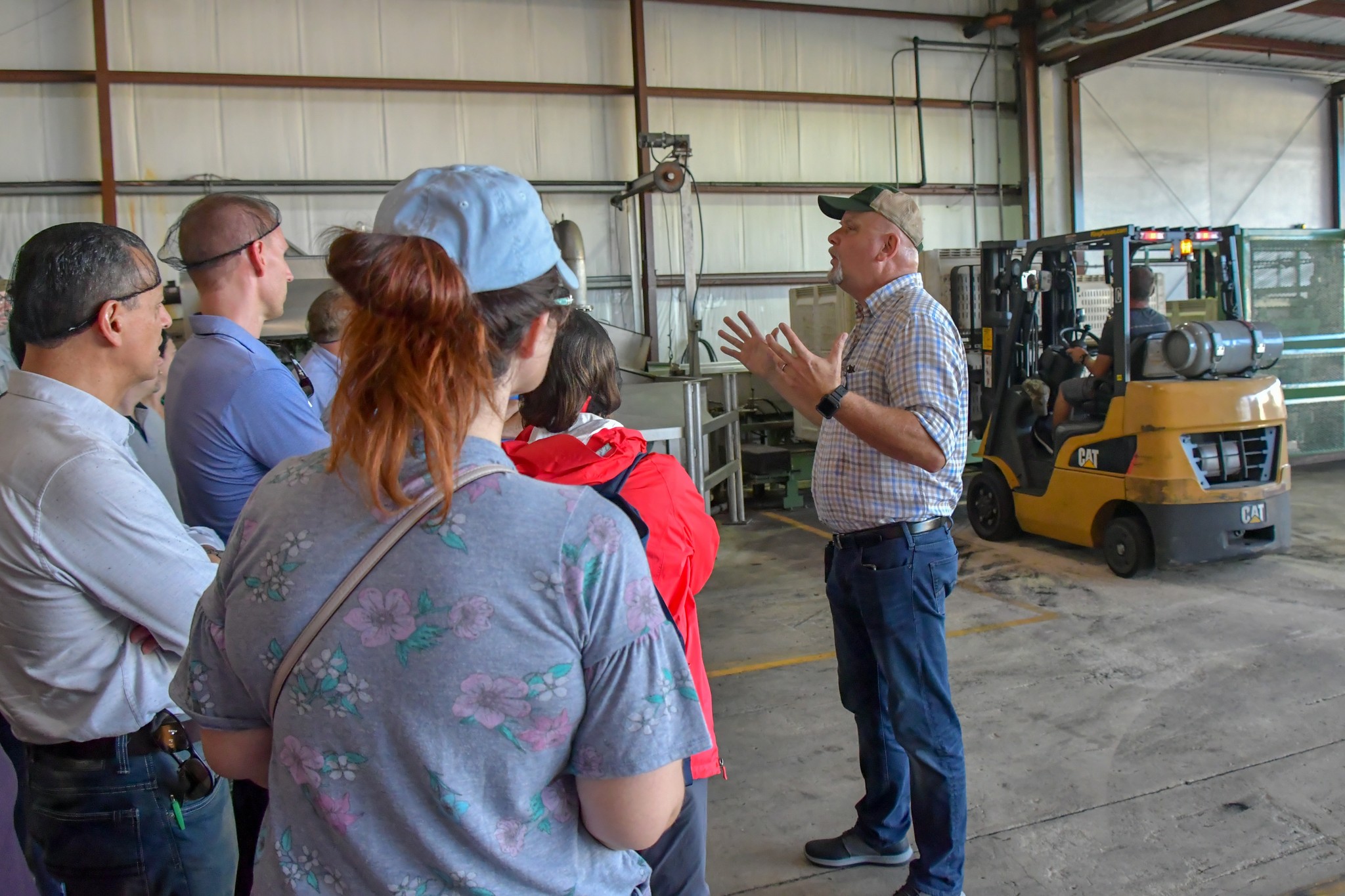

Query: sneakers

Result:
[894,883,968,896]
[1033,422,1054,455]
[804,827,912,868]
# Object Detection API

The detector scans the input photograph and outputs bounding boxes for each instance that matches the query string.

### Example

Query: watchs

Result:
[1079,352,1089,364]
[815,385,849,419]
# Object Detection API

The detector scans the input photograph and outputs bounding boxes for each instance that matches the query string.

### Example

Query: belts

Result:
[37,718,203,760]
[833,517,949,549]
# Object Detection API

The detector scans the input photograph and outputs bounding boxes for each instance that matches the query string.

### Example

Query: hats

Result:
[371,165,580,295]
[817,183,924,254]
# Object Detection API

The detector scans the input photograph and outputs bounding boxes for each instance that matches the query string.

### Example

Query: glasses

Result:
[258,338,314,399]
[149,708,214,801]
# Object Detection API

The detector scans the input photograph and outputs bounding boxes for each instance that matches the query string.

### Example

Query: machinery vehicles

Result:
[958,220,1297,579]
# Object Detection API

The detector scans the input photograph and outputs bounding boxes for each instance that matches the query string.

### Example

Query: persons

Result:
[2,220,240,896]
[0,274,20,400]
[716,184,970,895]
[1034,265,1171,453]
[295,290,354,446]
[167,191,334,546]
[114,350,187,526]
[167,163,712,895]
[500,304,724,896]
[135,330,180,453]
